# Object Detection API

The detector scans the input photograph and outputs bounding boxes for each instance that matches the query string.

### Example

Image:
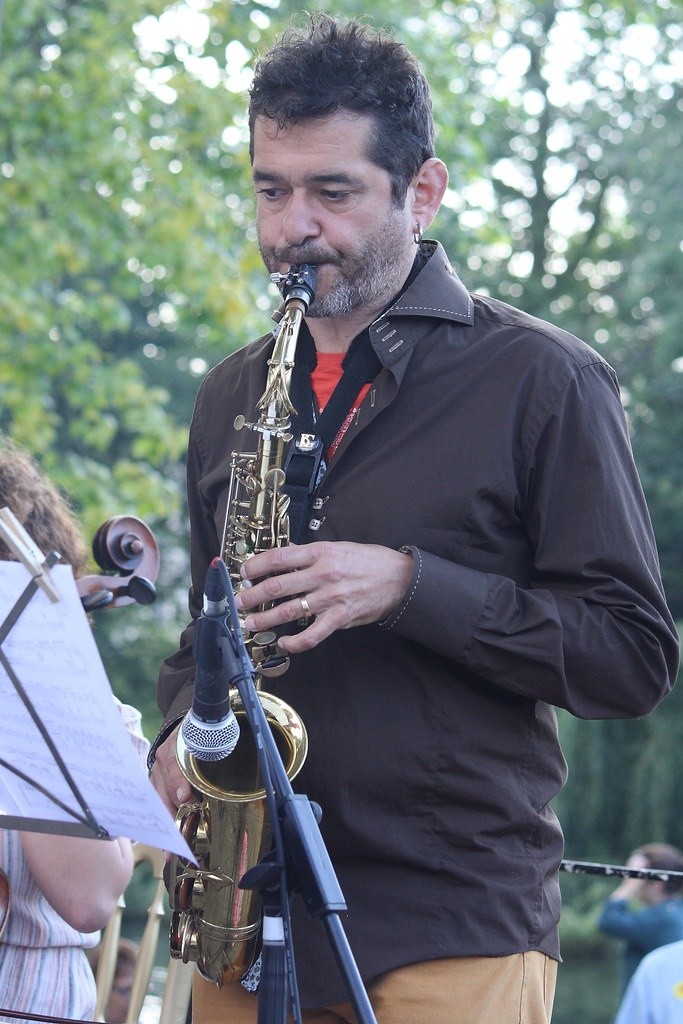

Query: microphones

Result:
[181,558,241,762]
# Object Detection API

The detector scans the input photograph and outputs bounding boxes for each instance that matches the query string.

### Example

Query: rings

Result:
[299,594,312,618]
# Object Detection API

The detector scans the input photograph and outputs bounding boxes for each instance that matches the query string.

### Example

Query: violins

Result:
[75,514,159,612]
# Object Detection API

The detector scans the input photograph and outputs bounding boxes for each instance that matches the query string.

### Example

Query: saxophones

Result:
[163,261,322,991]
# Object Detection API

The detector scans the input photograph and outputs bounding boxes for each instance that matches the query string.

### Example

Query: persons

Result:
[0,433,153,1024]
[596,842,683,1023]
[147,11,681,1023]
